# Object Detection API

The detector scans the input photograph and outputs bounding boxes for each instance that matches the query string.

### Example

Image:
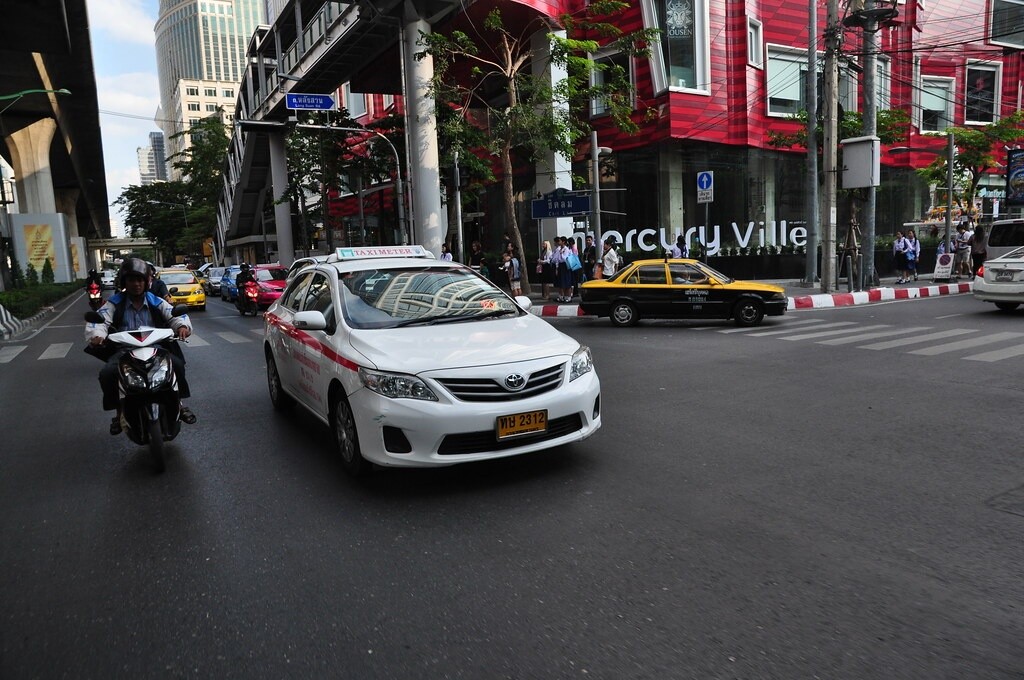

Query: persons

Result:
[550,235,623,303]
[440,242,453,262]
[673,269,708,285]
[235,262,260,310]
[497,232,522,303]
[83,257,197,436]
[892,230,921,285]
[83,268,106,307]
[469,241,490,282]
[930,222,988,284]
[536,240,557,300]
[672,234,687,260]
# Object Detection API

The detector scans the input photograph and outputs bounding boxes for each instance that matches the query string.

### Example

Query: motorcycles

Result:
[84,279,105,311]
[148,287,178,302]
[83,304,189,449]
[235,278,259,316]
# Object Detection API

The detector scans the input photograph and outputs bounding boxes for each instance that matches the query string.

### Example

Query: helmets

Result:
[89,269,97,275]
[240,263,249,270]
[119,258,148,287]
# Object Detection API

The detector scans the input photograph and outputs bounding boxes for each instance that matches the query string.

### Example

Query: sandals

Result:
[110,417,122,435]
[179,407,197,425]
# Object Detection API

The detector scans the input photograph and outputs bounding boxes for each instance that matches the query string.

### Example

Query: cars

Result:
[192,267,231,296]
[156,271,206,310]
[96,271,116,289]
[262,245,602,467]
[972,246,1024,310]
[577,249,789,327]
[220,265,256,303]
[283,254,393,307]
[245,261,295,303]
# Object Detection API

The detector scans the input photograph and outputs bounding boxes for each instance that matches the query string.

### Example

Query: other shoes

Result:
[956,271,973,279]
[914,274,918,281]
[895,279,900,284]
[906,279,910,282]
[899,280,906,284]
[553,296,572,303]
[542,294,550,300]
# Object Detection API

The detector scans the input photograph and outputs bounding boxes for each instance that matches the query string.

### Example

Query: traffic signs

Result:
[697,171,713,203]
[450,212,485,234]
[285,93,338,110]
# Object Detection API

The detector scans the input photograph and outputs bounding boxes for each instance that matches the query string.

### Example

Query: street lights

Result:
[147,201,188,228]
[888,146,953,254]
[591,147,612,260]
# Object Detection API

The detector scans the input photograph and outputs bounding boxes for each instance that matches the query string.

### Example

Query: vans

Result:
[984,218,1024,260]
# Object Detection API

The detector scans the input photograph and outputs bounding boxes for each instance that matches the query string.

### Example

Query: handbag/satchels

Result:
[535,264,543,274]
[565,252,582,271]
